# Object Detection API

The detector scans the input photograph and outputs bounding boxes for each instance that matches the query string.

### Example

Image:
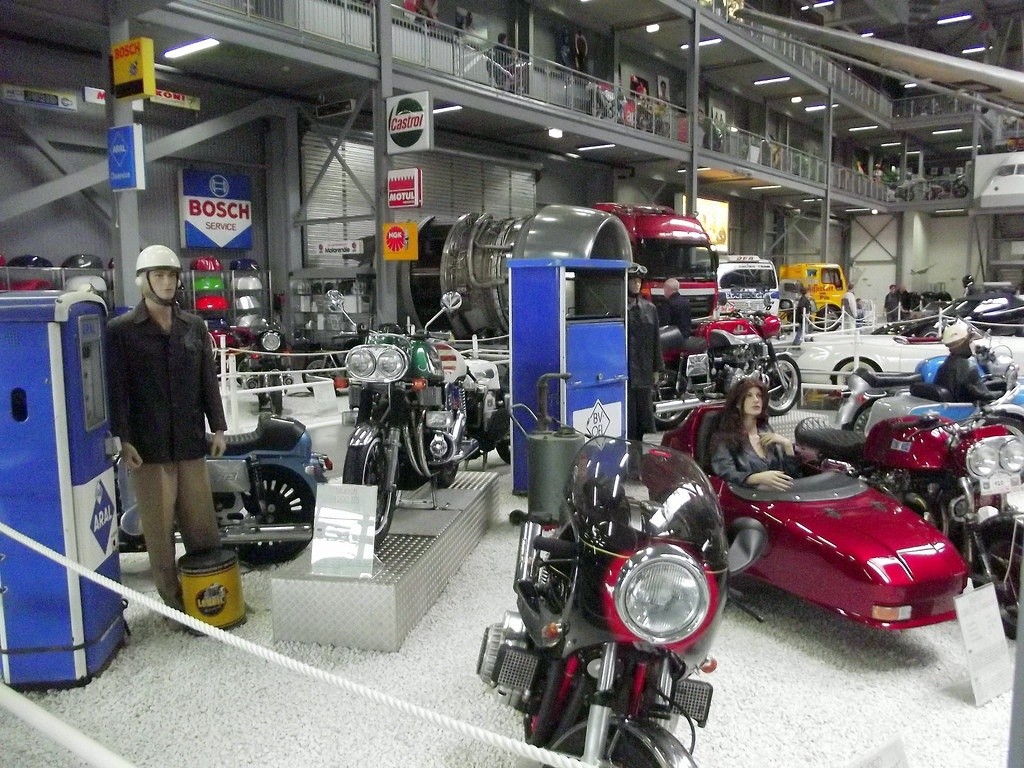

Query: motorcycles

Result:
[304,339,361,393]
[477,372,767,767]
[115,413,333,565]
[222,313,294,419]
[891,166,968,202]
[794,293,1024,639]
[325,290,511,551]
[651,293,801,431]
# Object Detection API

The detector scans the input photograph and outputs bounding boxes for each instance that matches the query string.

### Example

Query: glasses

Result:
[629,266,648,273]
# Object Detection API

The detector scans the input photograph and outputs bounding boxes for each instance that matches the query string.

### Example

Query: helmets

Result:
[628,262,644,281]
[136,245,181,273]
[941,318,976,353]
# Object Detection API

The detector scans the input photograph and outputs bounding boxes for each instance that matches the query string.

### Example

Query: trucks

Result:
[717,254,847,331]
[591,204,720,365]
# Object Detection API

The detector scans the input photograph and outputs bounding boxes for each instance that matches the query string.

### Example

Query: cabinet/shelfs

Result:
[176,268,274,329]
[289,266,377,345]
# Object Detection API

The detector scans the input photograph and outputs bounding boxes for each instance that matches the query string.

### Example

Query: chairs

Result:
[911,382,957,403]
[695,408,725,476]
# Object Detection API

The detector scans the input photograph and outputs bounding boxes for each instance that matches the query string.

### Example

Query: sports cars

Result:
[640,404,967,631]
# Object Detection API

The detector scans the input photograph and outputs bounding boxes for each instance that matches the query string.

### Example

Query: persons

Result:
[760,132,808,178]
[961,273,981,297]
[104,245,251,627]
[841,284,864,330]
[574,26,588,70]
[708,377,803,492]
[791,286,812,351]
[658,278,694,399]
[691,108,722,152]
[486,32,525,93]
[628,261,666,480]
[678,104,687,142]
[933,317,1004,406]
[883,283,911,323]
[661,79,669,103]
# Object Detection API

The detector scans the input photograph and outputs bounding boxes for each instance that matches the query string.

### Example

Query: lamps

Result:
[164,36,221,60]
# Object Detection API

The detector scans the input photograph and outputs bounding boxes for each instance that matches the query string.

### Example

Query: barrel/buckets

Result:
[177,551,246,635]
